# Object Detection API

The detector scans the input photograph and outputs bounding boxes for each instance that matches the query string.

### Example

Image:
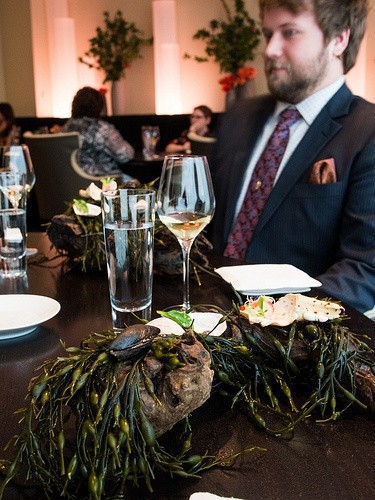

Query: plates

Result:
[0,294,62,340]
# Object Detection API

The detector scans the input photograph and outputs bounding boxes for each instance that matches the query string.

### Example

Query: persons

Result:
[0,102,21,146]
[165,106,217,162]
[192,0,375,314]
[61,86,135,182]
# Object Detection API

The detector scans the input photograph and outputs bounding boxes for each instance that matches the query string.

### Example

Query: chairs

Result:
[22,129,122,219]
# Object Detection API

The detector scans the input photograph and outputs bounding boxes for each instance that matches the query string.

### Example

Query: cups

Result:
[0,209,27,277]
[100,188,156,330]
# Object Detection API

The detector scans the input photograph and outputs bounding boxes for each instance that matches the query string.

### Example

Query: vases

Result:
[240,79,254,97]
[111,81,122,115]
[225,89,237,111]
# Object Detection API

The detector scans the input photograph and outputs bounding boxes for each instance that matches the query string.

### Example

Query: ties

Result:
[221,108,302,263]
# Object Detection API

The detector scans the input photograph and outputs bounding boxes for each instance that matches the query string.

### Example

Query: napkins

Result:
[146,311,228,337]
[212,264,323,296]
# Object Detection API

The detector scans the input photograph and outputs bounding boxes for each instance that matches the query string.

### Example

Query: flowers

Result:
[78,8,153,96]
[183,0,262,92]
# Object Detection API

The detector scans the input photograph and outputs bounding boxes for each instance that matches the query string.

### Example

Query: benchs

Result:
[16,113,224,170]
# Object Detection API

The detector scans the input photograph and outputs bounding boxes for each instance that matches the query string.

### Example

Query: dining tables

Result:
[0,234,375,500]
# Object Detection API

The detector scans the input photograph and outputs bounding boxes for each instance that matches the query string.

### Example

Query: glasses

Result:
[190,114,206,120]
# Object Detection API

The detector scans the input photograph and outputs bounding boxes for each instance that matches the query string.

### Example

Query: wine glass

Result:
[156,155,215,318]
[142,127,159,158]
[0,144,38,257]
